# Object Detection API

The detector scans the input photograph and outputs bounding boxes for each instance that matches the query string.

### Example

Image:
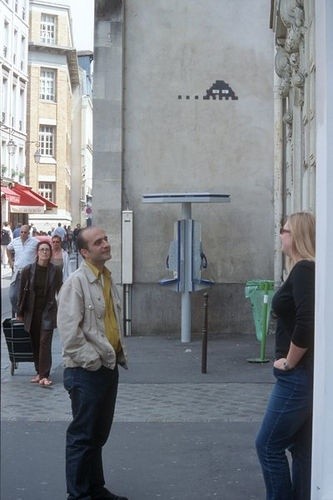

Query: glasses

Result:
[52,241,60,243]
[280,227,291,235]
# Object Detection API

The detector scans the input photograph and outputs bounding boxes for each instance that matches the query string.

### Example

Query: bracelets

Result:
[283,361,287,370]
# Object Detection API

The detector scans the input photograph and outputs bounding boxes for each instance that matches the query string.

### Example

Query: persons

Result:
[257,212,317,500]
[56,226,130,500]
[1,221,82,317]
[16,242,63,386]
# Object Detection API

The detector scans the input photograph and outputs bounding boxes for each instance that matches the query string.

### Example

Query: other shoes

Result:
[38,378,54,388]
[30,373,41,384]
